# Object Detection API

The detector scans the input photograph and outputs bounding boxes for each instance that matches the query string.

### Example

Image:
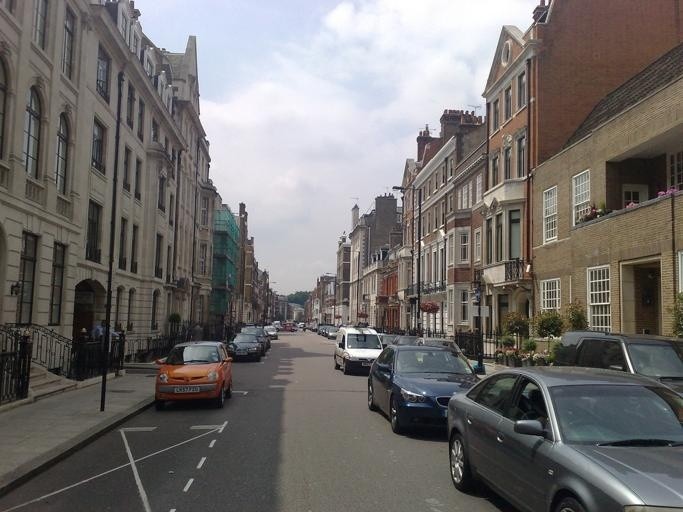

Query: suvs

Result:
[555,332,681,401]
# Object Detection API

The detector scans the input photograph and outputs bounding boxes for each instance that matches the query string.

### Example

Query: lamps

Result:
[9,281,20,297]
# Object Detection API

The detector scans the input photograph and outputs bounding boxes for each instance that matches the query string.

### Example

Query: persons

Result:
[78,328,90,343]
[91,319,121,342]
[110,326,124,358]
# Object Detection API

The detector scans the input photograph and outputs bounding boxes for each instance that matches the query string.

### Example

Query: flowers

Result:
[494,335,549,370]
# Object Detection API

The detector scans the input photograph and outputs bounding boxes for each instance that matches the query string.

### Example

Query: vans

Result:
[333,327,386,374]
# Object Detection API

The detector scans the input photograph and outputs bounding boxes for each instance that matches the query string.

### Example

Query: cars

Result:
[379,335,398,348]
[153,340,233,411]
[445,363,682,512]
[226,320,297,361]
[298,322,339,341]
[412,337,465,355]
[366,343,481,434]
[392,335,419,345]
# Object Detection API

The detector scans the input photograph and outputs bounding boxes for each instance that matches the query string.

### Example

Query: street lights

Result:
[391,186,421,331]
[468,279,487,374]
